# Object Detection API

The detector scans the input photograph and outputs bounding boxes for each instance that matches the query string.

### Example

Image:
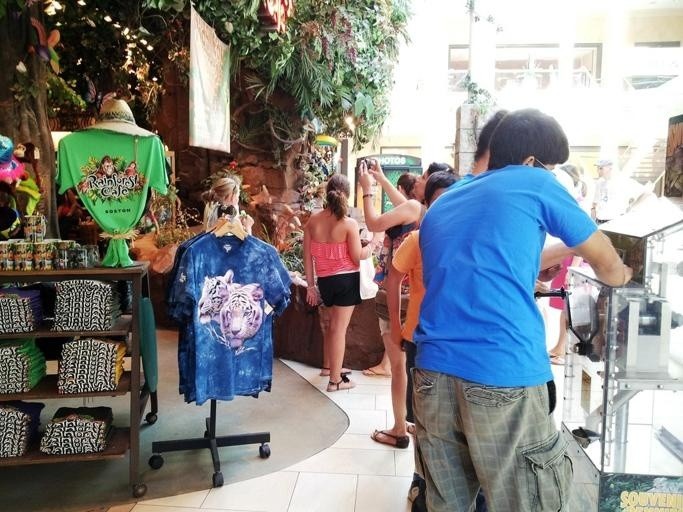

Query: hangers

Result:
[206,205,248,241]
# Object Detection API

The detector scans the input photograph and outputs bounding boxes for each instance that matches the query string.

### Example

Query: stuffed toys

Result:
[0,134,48,240]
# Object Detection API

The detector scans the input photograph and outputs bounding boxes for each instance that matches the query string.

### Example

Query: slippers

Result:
[364,368,385,375]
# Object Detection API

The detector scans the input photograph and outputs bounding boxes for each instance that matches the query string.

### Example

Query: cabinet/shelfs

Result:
[0,262,156,498]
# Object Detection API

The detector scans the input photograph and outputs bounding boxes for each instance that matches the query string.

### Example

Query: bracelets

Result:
[591,206,596,210]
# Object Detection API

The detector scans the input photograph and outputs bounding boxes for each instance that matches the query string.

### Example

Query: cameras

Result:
[364,158,371,171]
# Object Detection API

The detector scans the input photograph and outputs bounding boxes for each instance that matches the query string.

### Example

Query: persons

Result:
[409,108,634,510]
[534,161,590,363]
[419,110,574,510]
[202,176,253,239]
[56,182,81,236]
[357,153,463,447]
[304,175,378,392]
[592,152,617,226]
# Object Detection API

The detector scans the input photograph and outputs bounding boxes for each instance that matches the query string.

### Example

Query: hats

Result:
[595,161,613,168]
[84,99,155,137]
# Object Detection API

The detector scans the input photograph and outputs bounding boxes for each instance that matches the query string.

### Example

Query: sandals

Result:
[320,367,336,391]
[371,431,409,448]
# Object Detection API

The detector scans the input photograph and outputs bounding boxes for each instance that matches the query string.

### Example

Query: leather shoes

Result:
[549,350,564,365]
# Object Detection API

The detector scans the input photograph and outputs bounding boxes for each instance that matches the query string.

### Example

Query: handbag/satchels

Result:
[358,258,379,300]
[376,290,409,325]
